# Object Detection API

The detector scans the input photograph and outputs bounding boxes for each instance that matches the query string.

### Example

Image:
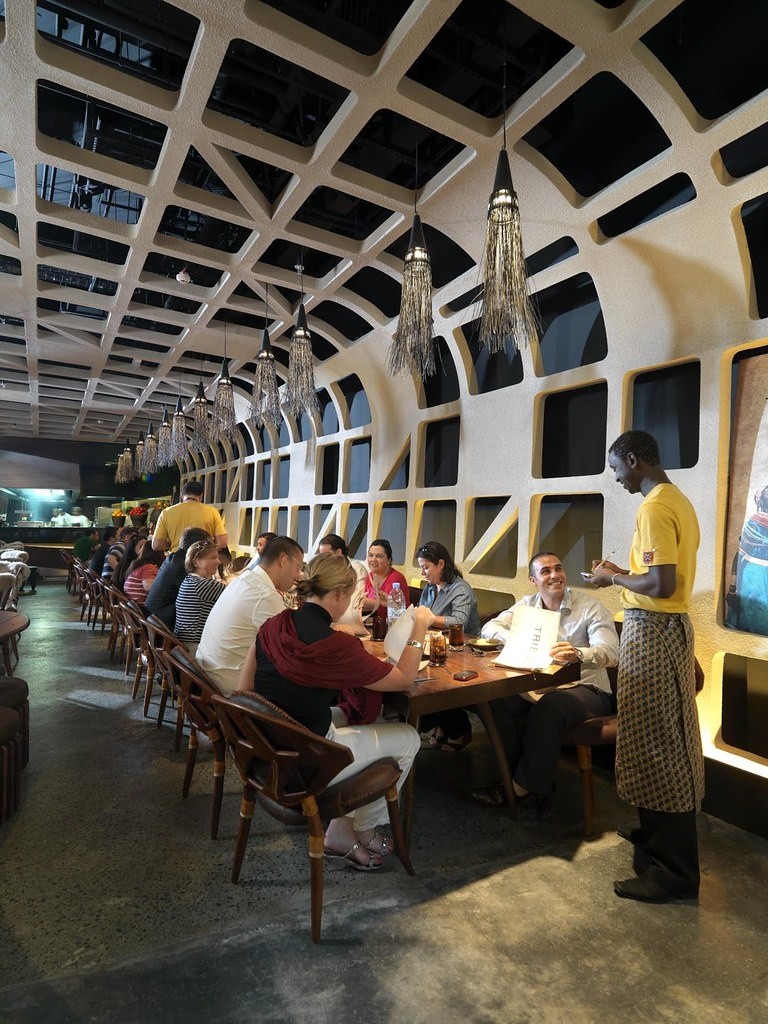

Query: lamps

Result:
[191,355,209,450]
[135,428,144,476]
[390,133,434,377]
[142,418,157,472]
[171,372,187,461]
[282,250,321,418]
[121,438,134,483]
[208,321,235,442]
[475,65,541,351]
[157,403,171,466]
[248,283,280,427]
[114,448,123,483]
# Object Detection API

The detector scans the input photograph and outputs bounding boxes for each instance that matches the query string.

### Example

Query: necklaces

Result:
[185,497,197,502]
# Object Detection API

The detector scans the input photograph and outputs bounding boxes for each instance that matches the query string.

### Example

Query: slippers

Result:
[359,829,394,856]
[322,843,383,870]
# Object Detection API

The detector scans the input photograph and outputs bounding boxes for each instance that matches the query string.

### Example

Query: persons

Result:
[152,481,228,556]
[143,527,482,872]
[579,430,705,904]
[49,506,165,614]
[473,551,621,818]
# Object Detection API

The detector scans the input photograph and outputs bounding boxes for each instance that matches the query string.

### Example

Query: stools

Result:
[0,676,29,824]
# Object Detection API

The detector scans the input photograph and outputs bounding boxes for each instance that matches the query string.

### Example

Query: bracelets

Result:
[406,640,426,650]
[577,650,583,662]
[611,572,622,588]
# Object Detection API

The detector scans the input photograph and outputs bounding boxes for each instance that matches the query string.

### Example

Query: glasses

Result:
[419,547,435,555]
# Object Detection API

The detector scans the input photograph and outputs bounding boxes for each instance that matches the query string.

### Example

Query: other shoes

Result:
[617,823,647,850]
[613,875,688,903]
[420,726,437,740]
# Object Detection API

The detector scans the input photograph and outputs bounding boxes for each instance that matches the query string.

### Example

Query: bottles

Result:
[387,583,406,632]
[89,520,92,527]
[95,516,99,528]
[51,521,55,527]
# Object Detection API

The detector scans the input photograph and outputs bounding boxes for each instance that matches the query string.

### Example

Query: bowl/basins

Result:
[469,638,503,650]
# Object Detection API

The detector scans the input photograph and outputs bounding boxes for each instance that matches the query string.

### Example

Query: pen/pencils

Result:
[591,550,616,574]
[414,676,440,682]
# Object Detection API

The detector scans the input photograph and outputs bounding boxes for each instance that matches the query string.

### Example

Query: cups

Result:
[371,615,388,642]
[392,609,408,626]
[429,639,447,667]
[449,624,464,652]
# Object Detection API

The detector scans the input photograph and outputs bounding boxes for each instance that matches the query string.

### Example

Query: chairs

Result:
[0,541,30,637]
[58,549,416,943]
[578,620,705,834]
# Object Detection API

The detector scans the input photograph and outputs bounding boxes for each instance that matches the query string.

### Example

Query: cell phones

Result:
[453,670,478,681]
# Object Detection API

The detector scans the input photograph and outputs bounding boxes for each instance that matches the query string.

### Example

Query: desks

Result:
[0,611,30,676]
[214,575,582,857]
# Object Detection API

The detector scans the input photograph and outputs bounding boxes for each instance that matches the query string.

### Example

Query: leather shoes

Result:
[471,773,532,806]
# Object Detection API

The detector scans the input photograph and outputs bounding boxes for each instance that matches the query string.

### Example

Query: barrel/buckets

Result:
[130,514,148,526]
[150,509,164,527]
[112,516,126,527]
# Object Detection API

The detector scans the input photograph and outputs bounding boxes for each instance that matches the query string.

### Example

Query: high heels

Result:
[420,726,446,748]
[442,730,471,756]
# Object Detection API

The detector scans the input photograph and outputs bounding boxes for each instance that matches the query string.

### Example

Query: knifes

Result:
[414,676,440,681]
